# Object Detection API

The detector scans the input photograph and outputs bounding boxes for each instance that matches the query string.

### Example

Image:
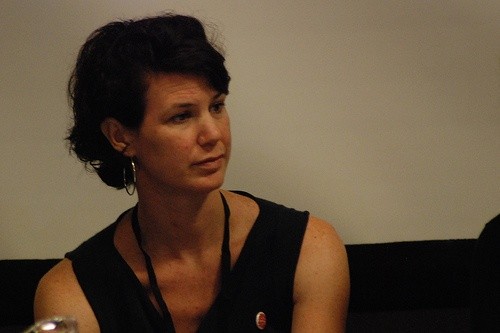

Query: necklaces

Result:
[130,189,232,333]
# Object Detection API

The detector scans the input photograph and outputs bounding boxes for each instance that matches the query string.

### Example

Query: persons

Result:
[34,11,353,333]
